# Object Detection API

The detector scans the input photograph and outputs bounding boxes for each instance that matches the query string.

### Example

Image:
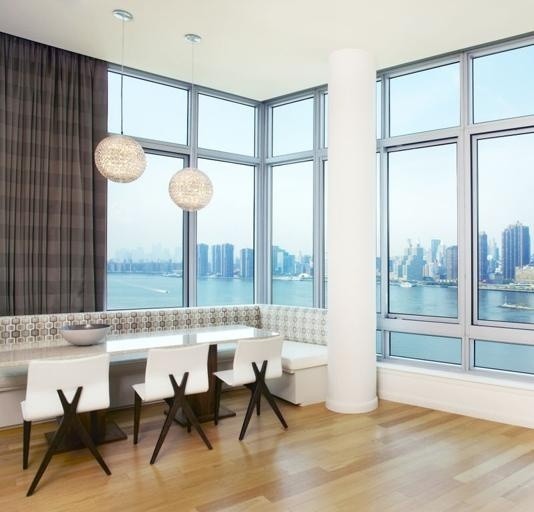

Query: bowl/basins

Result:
[58,323,111,346]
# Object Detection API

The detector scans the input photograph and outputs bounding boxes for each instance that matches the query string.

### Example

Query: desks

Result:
[0,326,279,453]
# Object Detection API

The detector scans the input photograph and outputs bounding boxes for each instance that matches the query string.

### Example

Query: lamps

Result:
[94,11,147,181]
[169,33,213,210]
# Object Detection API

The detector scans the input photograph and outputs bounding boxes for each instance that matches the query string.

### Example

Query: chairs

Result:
[213,333,288,440]
[21,353,117,496]
[131,343,213,465]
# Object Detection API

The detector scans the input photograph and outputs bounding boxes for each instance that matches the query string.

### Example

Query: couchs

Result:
[0,304,327,431]
